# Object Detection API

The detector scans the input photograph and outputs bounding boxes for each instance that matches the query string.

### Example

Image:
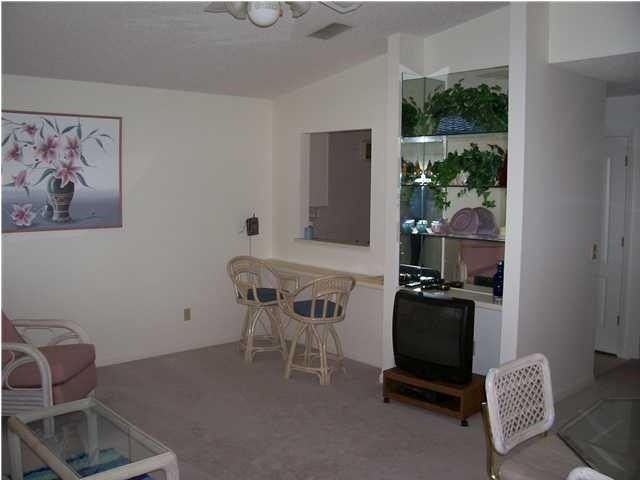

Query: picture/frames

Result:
[1,109,123,234]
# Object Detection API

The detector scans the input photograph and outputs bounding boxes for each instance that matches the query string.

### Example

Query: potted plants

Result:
[423,141,508,210]
[425,76,507,134]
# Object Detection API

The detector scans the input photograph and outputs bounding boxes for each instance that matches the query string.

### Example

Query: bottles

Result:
[493,261,503,297]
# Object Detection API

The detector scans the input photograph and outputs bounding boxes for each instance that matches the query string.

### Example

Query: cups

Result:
[402,222,440,235]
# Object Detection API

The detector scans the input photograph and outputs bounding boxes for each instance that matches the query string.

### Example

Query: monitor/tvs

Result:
[392,290,476,388]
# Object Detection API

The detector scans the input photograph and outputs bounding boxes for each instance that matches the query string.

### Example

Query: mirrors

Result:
[400,72,445,287]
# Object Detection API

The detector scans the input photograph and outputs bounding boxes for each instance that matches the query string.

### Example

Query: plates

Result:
[448,207,479,235]
[472,207,496,235]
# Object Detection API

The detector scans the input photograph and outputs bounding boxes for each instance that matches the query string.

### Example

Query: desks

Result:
[381,365,487,426]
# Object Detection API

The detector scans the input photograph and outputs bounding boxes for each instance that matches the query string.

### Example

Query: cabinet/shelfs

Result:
[402,64,510,309]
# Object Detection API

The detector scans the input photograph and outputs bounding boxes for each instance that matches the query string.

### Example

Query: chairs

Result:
[482,353,638,480]
[226,253,354,386]
[2,307,99,437]
